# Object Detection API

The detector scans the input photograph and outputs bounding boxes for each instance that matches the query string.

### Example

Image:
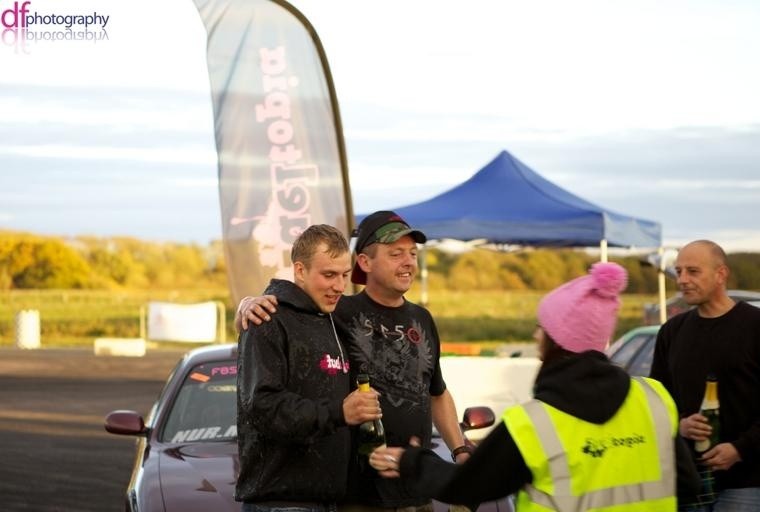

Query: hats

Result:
[355,210,428,255]
[535,261,630,355]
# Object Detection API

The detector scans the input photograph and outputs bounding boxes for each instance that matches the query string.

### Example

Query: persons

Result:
[233,212,760,512]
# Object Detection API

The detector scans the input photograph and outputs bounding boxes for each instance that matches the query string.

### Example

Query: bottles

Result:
[695,379,721,462]
[356,374,388,479]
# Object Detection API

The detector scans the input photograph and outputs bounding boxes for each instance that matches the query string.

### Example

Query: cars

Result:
[104,289,760,512]
